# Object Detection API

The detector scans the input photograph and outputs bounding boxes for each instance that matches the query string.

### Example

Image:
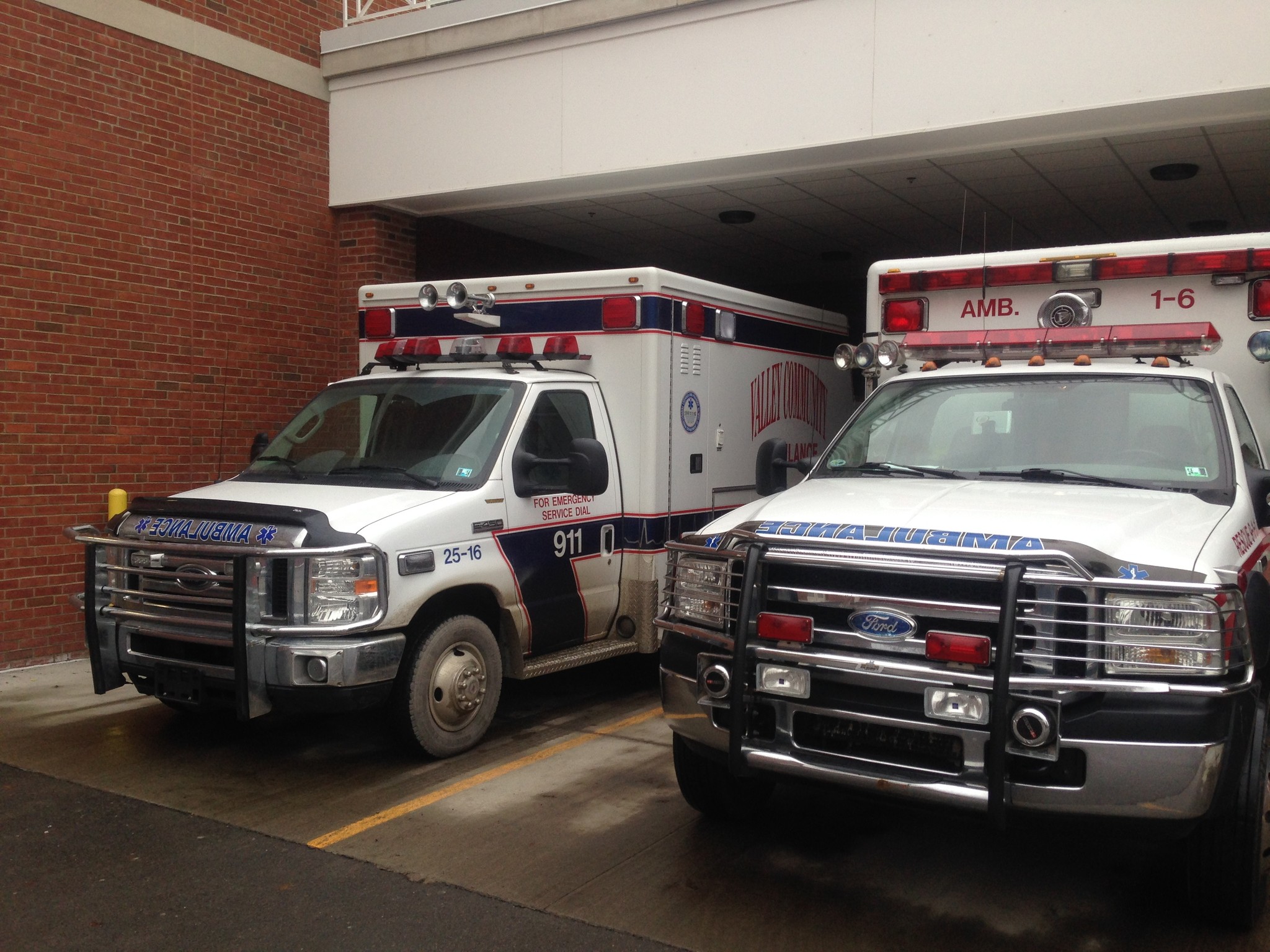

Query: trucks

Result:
[653,232,1270,906]
[62,266,853,760]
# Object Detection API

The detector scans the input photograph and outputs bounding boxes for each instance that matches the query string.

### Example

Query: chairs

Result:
[372,398,428,468]
[1128,426,1208,471]
[944,426,1014,469]
